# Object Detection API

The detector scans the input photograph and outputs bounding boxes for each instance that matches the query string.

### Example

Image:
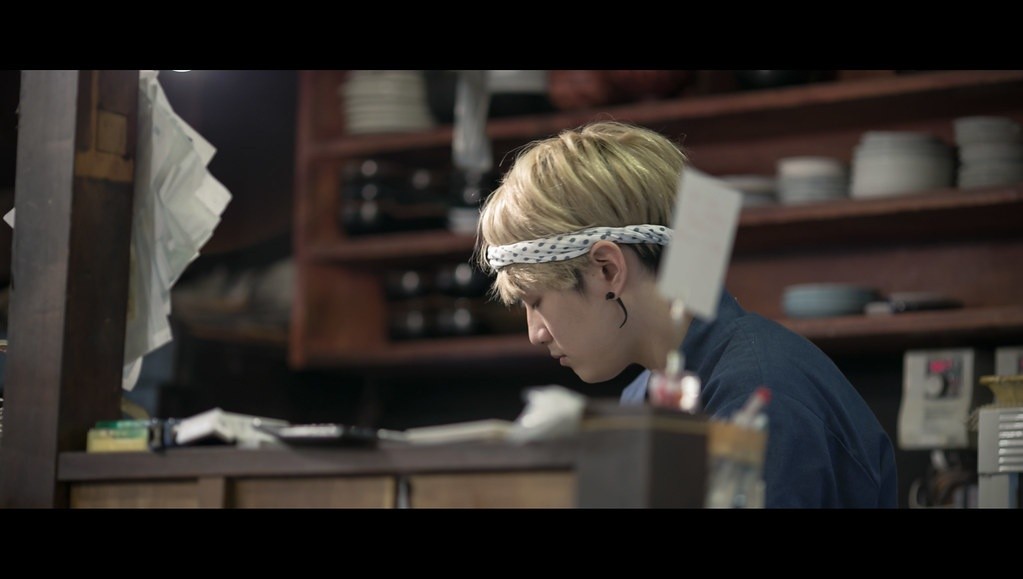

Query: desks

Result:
[55,433,768,508]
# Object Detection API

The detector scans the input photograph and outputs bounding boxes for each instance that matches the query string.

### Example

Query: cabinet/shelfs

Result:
[289,70,1023,374]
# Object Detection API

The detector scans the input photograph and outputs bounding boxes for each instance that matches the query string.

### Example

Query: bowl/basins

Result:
[337,157,451,236]
[780,281,871,316]
[383,253,493,335]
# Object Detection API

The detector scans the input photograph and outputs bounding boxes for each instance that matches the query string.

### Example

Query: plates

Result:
[953,115,1022,190]
[775,155,848,204]
[339,69,438,136]
[718,174,776,205]
[847,130,954,199]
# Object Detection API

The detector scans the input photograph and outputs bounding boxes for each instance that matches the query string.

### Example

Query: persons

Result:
[472,121,902,508]
